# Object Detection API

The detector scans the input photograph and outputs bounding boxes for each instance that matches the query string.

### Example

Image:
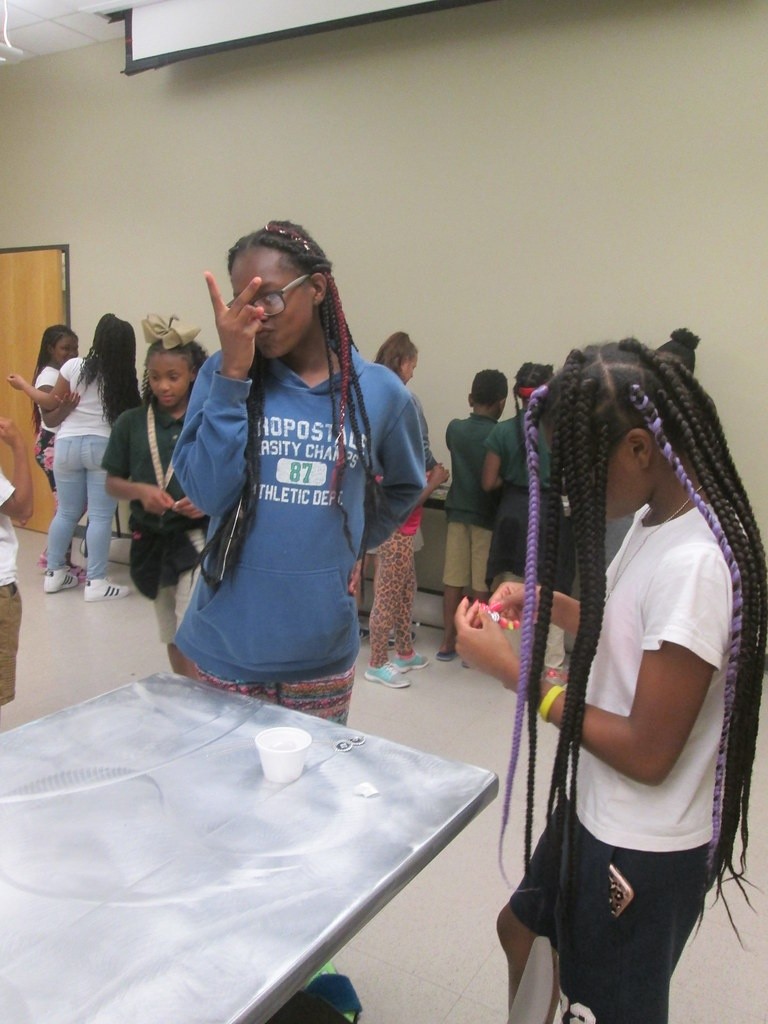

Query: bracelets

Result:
[541,685,566,724]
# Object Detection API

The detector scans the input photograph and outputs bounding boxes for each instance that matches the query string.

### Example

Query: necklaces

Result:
[605,485,704,603]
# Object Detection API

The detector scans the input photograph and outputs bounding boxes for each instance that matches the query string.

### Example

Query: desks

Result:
[0,668,500,1024]
[356,484,573,630]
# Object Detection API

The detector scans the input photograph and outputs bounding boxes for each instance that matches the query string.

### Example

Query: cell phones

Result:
[608,863,633,918]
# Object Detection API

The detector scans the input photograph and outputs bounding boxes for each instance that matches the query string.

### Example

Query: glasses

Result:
[225,273,311,316]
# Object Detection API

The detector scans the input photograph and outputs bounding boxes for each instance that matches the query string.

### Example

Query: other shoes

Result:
[386,631,416,650]
[359,629,371,640]
[38,554,86,577]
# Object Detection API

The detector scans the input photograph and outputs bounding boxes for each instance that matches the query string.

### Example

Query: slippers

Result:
[435,651,473,670]
[302,969,362,1024]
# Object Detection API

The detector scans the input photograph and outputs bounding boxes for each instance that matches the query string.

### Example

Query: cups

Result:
[255,726,313,784]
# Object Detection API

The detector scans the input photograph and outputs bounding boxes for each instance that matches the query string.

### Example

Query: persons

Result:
[349,332,450,688]
[172,220,427,726]
[100,313,210,681]
[654,329,700,376]
[7,313,142,601]
[454,338,768,1024]
[0,416,34,722]
[435,364,555,666]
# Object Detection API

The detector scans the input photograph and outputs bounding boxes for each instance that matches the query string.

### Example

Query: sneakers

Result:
[364,661,411,688]
[84,578,130,602]
[44,570,79,593]
[393,650,430,672]
